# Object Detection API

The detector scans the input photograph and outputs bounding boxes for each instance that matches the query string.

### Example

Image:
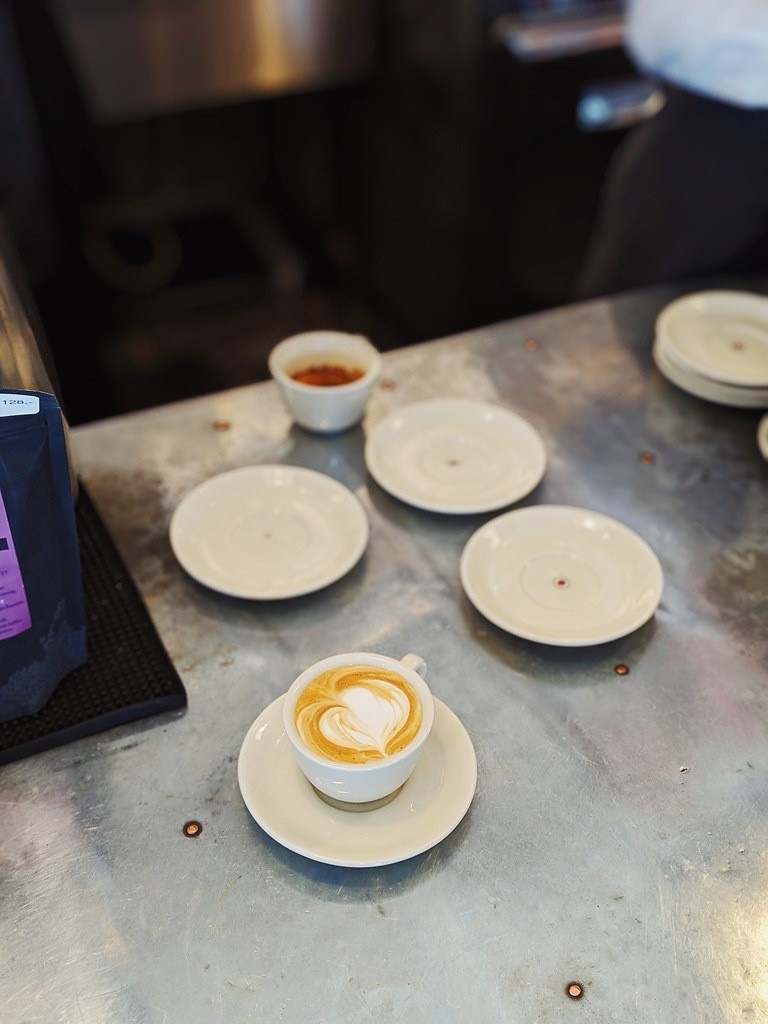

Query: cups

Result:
[269,331,382,435]
[282,653,435,803]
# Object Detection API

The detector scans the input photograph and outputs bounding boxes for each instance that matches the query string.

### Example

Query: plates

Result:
[364,398,545,514]
[652,290,768,462]
[461,503,665,646]
[169,465,372,600]
[236,692,476,867]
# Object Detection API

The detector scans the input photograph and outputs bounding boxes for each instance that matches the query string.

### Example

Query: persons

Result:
[572,4,768,297]
